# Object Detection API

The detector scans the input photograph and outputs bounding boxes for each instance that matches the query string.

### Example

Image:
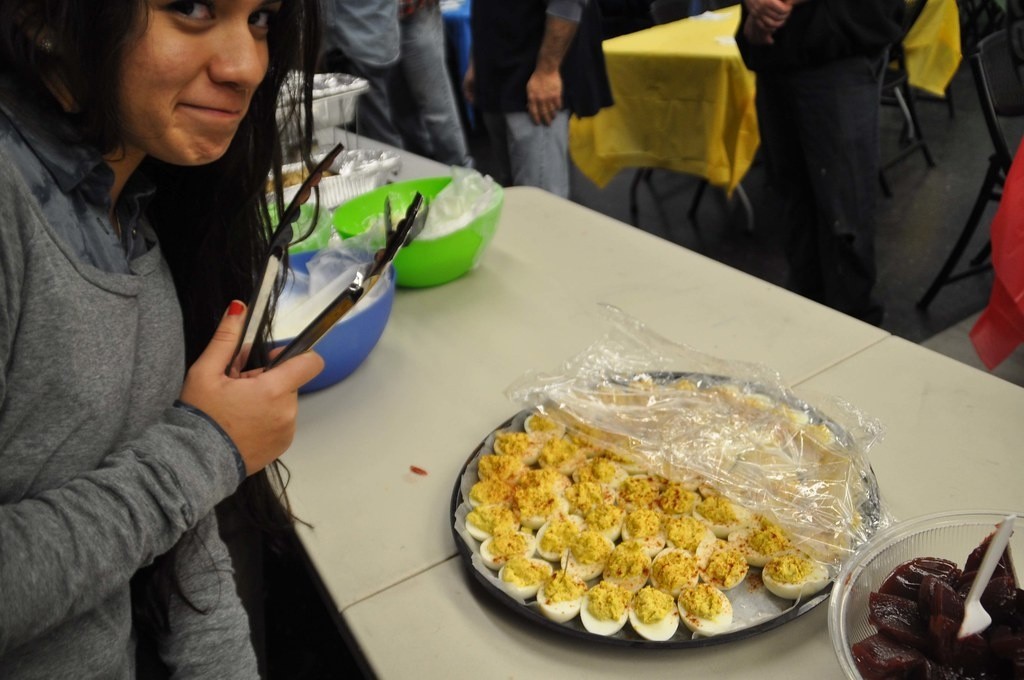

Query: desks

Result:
[568,0,962,241]
[266,117,1024,680]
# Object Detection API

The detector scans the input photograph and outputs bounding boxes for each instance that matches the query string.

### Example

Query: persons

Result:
[318,0,907,326]
[0,0,325,680]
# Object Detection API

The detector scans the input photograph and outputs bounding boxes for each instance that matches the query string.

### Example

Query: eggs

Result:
[464,378,864,641]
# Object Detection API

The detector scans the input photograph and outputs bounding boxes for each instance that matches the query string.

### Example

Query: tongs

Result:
[222,143,423,377]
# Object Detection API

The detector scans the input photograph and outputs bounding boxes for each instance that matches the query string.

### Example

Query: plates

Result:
[450,370,880,649]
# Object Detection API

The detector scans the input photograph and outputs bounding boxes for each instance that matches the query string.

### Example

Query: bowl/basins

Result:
[269,148,402,207]
[277,67,370,131]
[264,245,396,394]
[828,509,1024,680]
[332,175,504,288]
[263,199,329,253]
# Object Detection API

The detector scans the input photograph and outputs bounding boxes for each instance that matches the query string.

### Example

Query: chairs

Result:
[852,1,939,173]
[916,28,1024,319]
